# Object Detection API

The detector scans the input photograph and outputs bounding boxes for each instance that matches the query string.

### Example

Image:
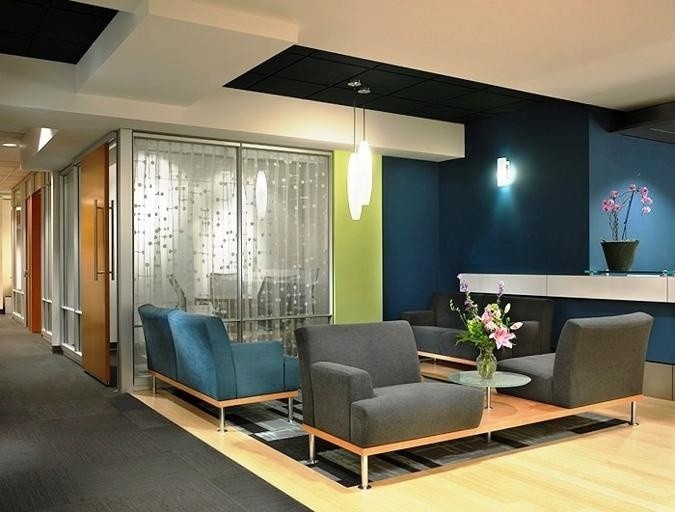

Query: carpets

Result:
[154,373,635,493]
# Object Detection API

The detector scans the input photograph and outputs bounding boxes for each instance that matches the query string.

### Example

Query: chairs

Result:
[166,268,320,317]
[495,314,655,430]
[296,320,490,487]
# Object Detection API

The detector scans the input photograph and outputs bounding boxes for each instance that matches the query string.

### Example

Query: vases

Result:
[600,240,640,272]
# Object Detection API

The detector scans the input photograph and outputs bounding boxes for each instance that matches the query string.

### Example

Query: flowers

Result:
[601,183,654,241]
[449,276,526,377]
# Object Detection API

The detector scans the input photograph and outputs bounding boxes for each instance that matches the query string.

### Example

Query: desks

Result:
[449,369,531,412]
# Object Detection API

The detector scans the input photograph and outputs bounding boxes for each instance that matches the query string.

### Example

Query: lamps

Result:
[346,81,375,223]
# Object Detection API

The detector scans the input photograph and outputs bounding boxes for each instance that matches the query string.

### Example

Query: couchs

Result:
[402,289,556,371]
[138,303,303,433]
